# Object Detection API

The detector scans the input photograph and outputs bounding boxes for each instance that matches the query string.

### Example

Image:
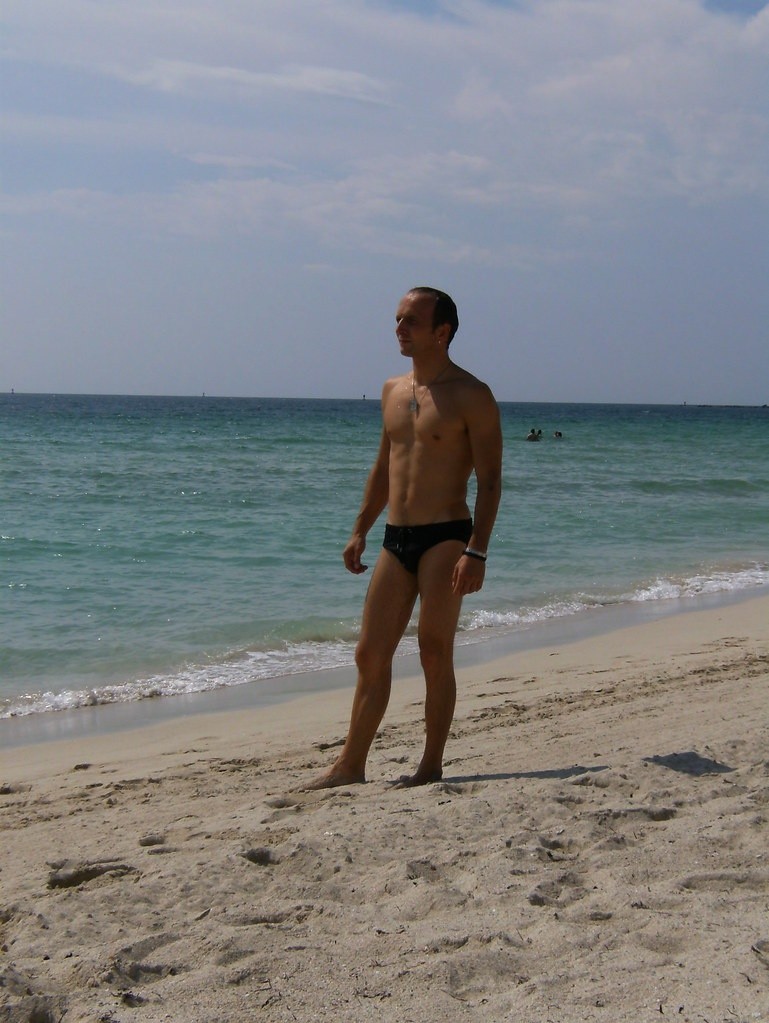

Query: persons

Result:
[303,286,502,790]
[528,429,562,441]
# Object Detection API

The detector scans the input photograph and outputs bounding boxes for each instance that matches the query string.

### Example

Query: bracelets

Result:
[462,548,487,561]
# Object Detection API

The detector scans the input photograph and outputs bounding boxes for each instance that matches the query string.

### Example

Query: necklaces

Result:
[409,360,452,412]
